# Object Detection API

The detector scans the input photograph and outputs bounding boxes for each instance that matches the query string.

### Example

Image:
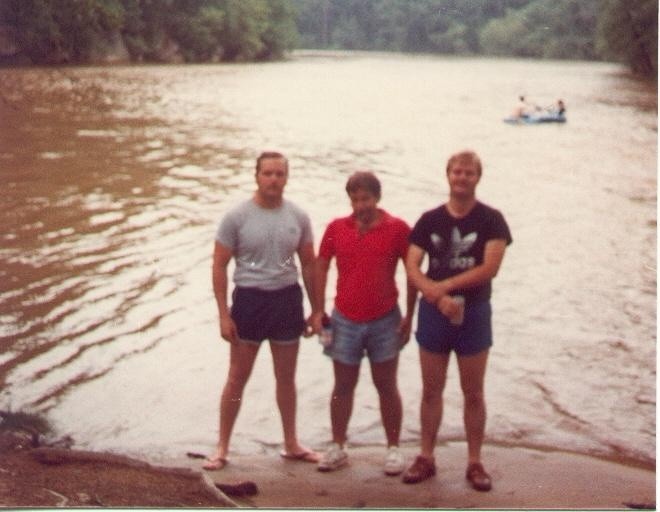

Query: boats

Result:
[503,111,567,125]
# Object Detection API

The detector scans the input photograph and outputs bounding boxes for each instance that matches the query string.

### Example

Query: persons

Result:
[203,150,321,468]
[548,99,567,117]
[397,148,516,492]
[511,95,530,118]
[322,168,420,474]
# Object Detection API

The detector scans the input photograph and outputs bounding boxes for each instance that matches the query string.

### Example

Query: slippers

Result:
[202,457,231,471]
[280,448,320,463]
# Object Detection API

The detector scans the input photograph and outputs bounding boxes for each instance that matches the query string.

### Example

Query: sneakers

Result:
[466,464,492,492]
[401,454,437,484]
[384,446,407,474]
[317,443,350,471]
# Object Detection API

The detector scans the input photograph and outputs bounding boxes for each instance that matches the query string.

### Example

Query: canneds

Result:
[451,295,465,325]
